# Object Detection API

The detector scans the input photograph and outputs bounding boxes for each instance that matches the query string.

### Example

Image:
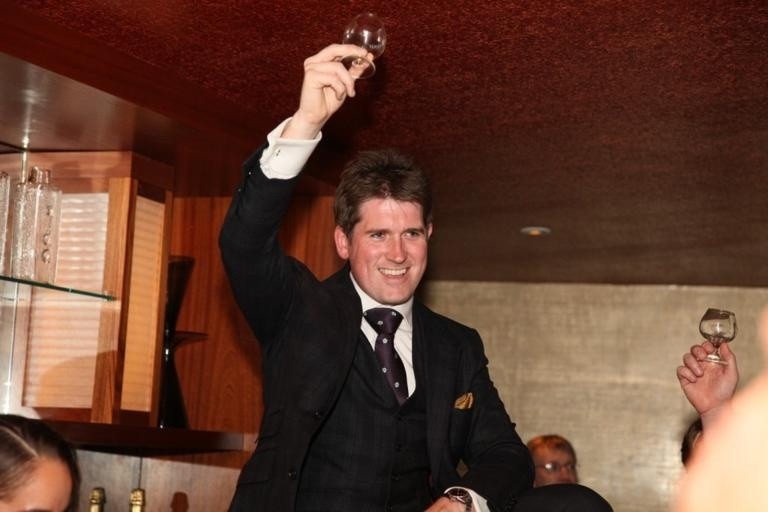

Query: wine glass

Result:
[698,307,738,366]
[340,11,389,78]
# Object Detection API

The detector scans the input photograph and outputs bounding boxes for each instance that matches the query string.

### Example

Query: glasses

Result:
[536,463,581,473]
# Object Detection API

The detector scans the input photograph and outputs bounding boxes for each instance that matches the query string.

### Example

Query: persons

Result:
[221,42,533,512]
[0,416,80,511]
[674,311,767,511]
[531,434,580,487]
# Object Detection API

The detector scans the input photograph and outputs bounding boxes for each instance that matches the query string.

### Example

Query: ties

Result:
[363,308,408,406]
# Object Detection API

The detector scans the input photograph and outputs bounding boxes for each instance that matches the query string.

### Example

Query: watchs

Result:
[443,488,472,512]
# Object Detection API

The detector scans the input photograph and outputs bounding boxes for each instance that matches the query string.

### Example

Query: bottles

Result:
[88,487,105,512]
[129,487,145,512]
[7,165,63,283]
